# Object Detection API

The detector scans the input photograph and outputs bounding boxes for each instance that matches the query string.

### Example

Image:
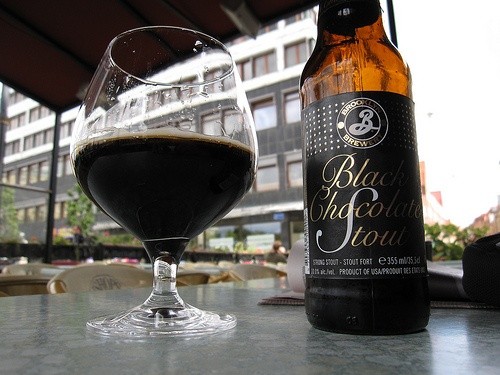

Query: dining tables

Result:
[0,257,499,373]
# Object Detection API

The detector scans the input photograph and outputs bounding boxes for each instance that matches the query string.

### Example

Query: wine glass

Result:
[69,23,260,340]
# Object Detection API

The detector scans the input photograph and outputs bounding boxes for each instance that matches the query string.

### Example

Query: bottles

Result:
[296,1,431,337]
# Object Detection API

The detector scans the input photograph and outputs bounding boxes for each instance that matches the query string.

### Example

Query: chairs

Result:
[0,255,288,297]
